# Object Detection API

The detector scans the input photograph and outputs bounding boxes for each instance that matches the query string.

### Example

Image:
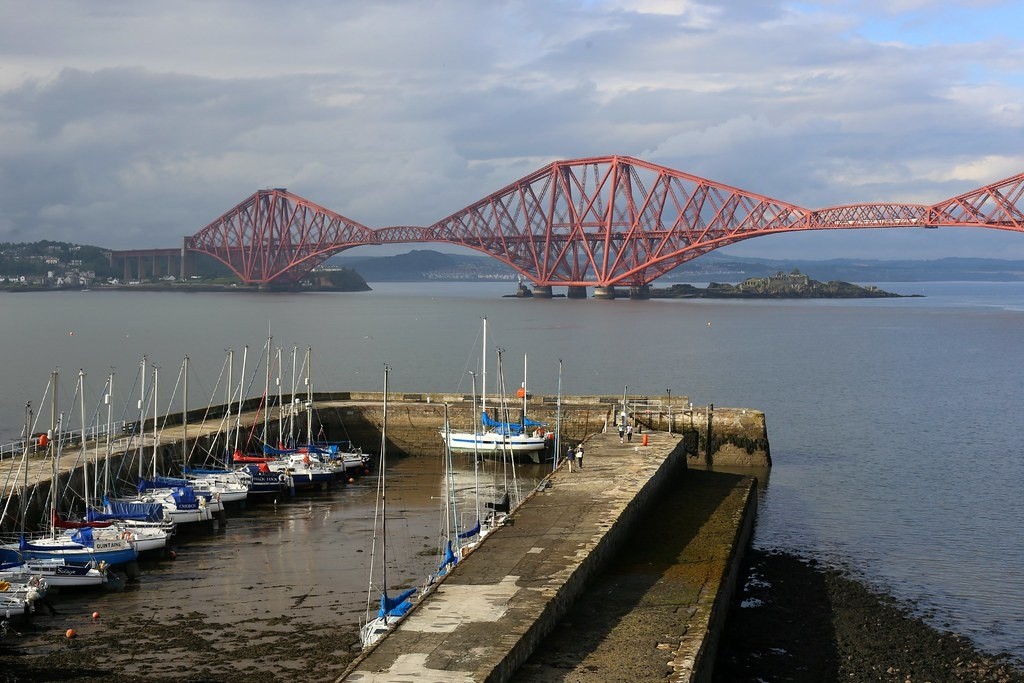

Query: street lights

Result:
[666,387,674,436]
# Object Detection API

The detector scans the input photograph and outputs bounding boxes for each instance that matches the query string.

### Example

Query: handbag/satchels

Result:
[576,452,583,458]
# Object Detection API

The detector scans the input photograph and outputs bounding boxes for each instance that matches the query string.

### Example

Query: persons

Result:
[567,446,576,473]
[618,419,624,444]
[577,444,584,469]
[626,423,632,441]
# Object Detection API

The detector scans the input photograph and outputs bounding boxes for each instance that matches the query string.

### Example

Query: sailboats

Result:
[1,322,367,640]
[436,316,554,464]
[427,318,526,587]
[357,362,440,654]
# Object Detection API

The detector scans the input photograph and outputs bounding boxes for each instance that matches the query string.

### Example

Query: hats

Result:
[578,444,585,448]
[568,446,572,449]
